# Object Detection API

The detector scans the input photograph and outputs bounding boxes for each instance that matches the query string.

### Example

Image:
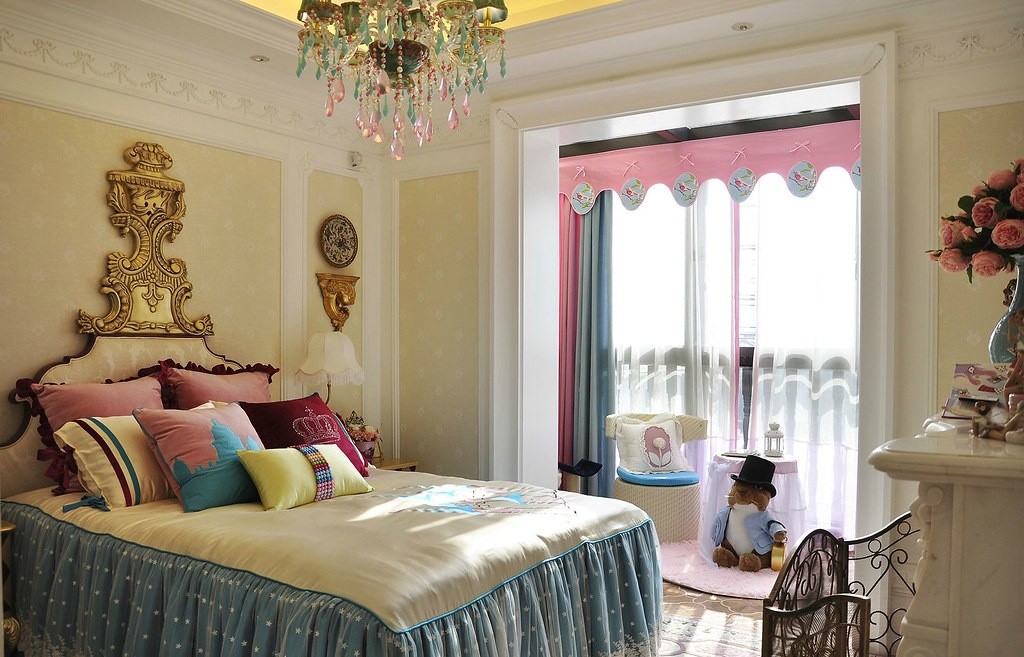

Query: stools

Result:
[698,448,806,572]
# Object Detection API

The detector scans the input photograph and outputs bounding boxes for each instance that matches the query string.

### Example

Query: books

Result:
[941,362,1017,418]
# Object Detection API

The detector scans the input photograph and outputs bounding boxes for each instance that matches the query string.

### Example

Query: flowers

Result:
[925,158,1024,285]
[345,410,385,462]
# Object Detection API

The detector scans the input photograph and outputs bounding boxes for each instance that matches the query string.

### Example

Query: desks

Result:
[867,410,1024,657]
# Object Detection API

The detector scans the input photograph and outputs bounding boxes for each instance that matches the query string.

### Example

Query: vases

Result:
[988,255,1024,395]
[356,441,375,463]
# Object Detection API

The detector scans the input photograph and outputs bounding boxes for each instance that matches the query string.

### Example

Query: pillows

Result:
[616,414,694,475]
[16,358,374,513]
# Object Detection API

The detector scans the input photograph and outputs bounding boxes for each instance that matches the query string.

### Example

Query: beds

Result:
[0,142,661,657]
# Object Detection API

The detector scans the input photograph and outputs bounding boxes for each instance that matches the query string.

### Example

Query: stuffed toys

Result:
[709,456,787,571]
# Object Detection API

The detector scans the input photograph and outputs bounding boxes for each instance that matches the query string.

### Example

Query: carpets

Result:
[660,540,854,600]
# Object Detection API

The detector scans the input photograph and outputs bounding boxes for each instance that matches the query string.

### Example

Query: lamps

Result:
[294,332,364,406]
[295,0,509,160]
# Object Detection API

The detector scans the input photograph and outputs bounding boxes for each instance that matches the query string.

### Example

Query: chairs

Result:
[606,413,708,543]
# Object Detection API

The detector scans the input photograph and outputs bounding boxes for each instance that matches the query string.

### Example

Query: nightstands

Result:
[371,455,418,472]
